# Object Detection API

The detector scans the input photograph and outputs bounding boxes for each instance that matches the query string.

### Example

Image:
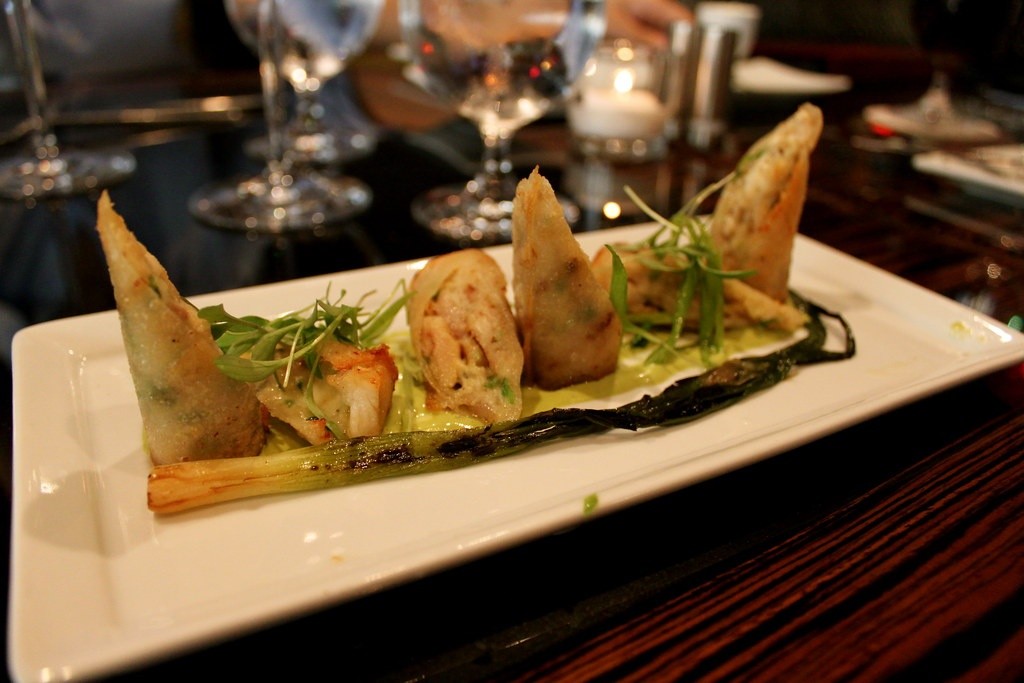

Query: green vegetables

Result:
[174,151,761,444]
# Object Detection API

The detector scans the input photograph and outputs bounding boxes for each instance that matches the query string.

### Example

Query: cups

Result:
[573,42,672,162]
[668,20,739,149]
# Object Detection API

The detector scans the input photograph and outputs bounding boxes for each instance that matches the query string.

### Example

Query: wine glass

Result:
[397,1,600,239]
[188,0,388,233]
[0,0,138,200]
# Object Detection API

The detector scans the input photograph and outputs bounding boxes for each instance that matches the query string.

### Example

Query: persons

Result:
[0,0,1024,135]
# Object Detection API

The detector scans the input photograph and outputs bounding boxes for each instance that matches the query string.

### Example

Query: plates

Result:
[7,216,1023,683]
[914,145,1024,207]
